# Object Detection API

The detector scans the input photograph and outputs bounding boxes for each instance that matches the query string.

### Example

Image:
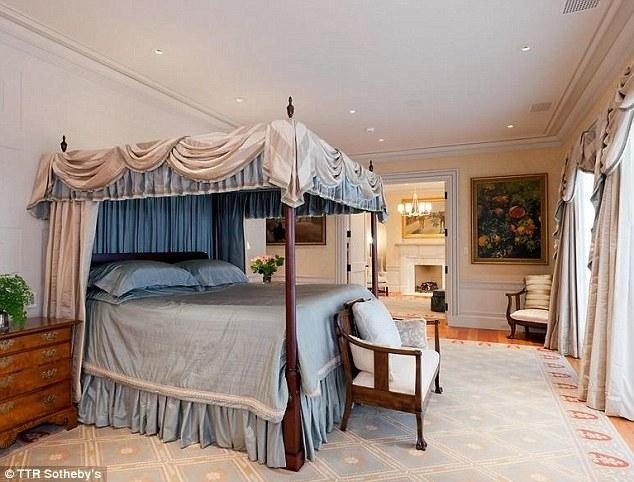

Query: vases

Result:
[262,273,271,282]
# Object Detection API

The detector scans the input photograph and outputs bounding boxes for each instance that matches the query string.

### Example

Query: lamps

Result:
[397,187,432,216]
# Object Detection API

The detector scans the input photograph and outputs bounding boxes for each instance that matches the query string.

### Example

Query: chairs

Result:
[505,274,553,339]
[334,311,443,451]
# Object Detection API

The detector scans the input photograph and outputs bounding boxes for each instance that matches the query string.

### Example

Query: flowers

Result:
[250,255,284,276]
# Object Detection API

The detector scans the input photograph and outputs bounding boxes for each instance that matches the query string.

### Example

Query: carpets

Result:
[19,339,634,482]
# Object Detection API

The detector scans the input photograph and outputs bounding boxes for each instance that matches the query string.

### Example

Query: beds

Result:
[26,96,388,472]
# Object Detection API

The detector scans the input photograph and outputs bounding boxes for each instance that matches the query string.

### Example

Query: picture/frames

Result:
[266,216,326,245]
[400,196,445,239]
[470,173,549,265]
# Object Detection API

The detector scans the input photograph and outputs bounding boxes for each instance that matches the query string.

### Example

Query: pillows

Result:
[88,259,248,305]
[348,334,393,381]
[395,320,427,349]
[352,298,402,350]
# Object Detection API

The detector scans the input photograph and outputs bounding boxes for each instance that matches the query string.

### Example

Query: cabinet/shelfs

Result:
[0,316,82,449]
